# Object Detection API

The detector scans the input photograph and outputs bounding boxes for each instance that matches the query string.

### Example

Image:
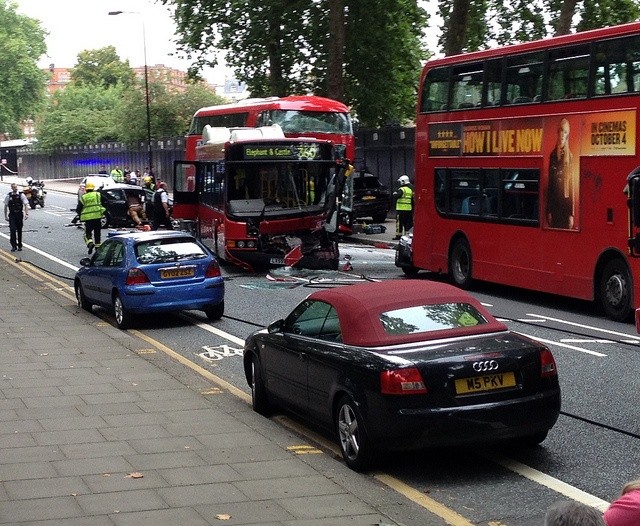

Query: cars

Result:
[78,174,111,197]
[97,183,174,227]
[341,170,390,223]
[242,282,562,474]
[71,228,229,330]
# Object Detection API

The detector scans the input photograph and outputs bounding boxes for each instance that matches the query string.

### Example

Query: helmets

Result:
[25,176,32,183]
[85,182,95,190]
[397,175,410,185]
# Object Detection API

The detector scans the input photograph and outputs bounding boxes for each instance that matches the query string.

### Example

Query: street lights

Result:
[107,8,156,178]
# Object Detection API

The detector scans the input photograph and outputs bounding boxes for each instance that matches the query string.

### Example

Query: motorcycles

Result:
[393,227,419,276]
[20,179,48,208]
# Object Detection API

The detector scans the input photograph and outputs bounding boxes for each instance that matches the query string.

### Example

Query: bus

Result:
[172,138,353,272]
[413,21,640,322]
[185,95,356,191]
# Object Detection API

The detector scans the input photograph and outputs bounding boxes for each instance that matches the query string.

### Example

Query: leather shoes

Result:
[11,247,17,252]
[17,246,23,251]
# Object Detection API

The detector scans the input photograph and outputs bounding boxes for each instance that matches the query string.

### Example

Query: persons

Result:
[149,167,155,184]
[123,165,132,177]
[392,174,414,240]
[156,177,162,188]
[602,478,640,525]
[142,167,148,179]
[123,174,135,185]
[545,117,575,228]
[546,498,603,526]
[69,184,86,228]
[142,174,156,191]
[128,197,146,226]
[98,165,108,177]
[130,169,141,181]
[110,164,124,182]
[76,182,106,255]
[150,182,173,229]
[3,183,28,252]
